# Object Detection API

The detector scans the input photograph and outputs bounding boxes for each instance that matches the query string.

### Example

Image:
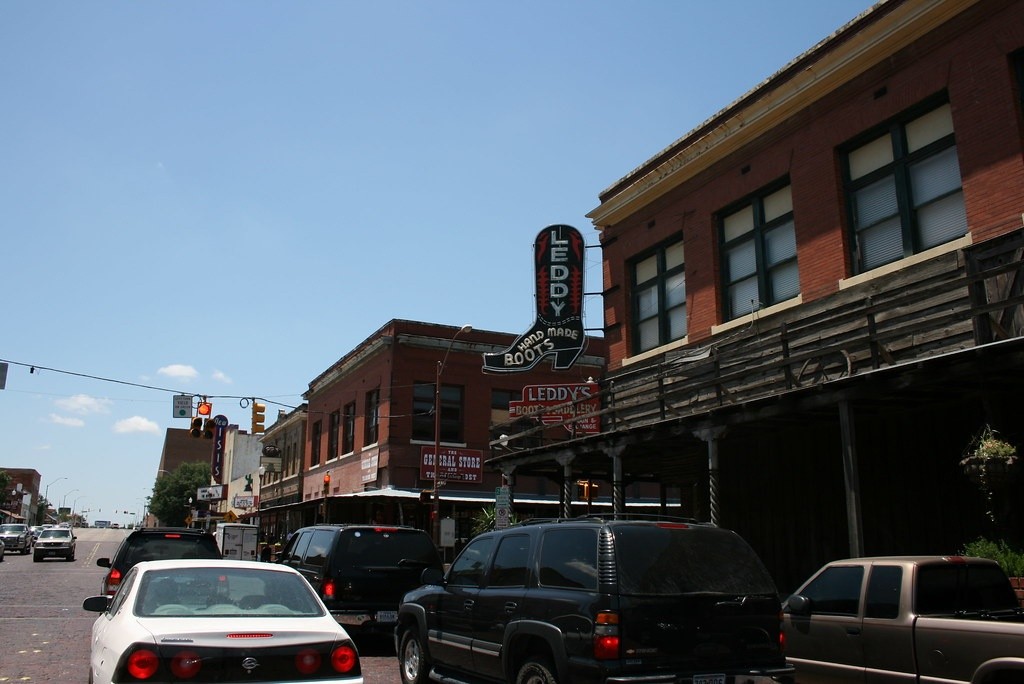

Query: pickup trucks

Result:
[782,555,1024,684]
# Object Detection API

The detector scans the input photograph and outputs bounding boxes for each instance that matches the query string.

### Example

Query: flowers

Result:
[960,423,1018,523]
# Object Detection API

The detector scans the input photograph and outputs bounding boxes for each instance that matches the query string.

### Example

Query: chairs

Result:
[238,594,269,610]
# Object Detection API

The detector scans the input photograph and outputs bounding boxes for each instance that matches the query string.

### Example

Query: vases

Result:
[960,455,1009,485]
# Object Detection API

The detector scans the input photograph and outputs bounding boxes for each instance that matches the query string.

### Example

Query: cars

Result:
[30,523,69,547]
[97,524,106,528]
[34,528,77,562]
[83,559,363,684]
[111,523,120,529]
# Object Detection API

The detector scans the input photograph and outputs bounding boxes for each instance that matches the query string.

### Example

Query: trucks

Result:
[213,522,258,561]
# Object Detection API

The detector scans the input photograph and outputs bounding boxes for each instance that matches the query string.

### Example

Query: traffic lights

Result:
[324,475,330,494]
[189,402,215,442]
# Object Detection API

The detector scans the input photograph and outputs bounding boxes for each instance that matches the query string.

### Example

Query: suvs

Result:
[394,512,795,684]
[0,524,32,562]
[96,528,230,617]
[278,522,445,627]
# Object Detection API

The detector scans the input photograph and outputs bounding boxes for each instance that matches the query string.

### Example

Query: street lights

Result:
[64,489,79,506]
[257,464,266,539]
[42,477,67,523]
[136,497,146,516]
[500,433,509,488]
[189,498,192,520]
[253,403,265,432]
[431,326,472,546]
